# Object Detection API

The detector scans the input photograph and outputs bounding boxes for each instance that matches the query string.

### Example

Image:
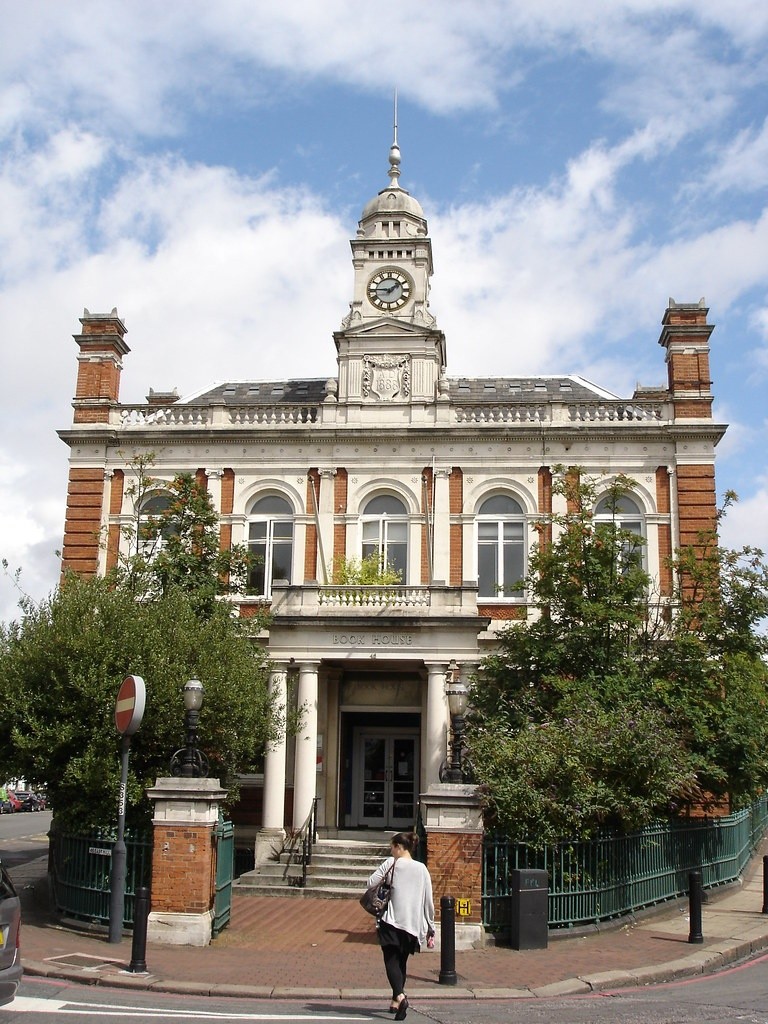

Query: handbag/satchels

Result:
[360,858,397,917]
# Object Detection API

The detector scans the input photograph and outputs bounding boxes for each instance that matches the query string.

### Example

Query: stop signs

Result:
[113,674,147,738]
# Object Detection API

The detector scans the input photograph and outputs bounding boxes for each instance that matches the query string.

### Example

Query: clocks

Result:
[366,268,412,312]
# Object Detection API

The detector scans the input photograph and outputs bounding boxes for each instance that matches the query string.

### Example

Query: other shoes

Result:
[390,1007,398,1013]
[395,999,408,1020]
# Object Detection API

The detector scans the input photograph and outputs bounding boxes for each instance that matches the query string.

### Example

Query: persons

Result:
[366,831,435,1021]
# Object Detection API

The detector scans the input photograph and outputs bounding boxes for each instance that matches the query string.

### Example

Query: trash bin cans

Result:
[511,869,549,949]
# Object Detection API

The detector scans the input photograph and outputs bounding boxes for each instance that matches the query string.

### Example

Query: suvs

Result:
[0,857,25,1007]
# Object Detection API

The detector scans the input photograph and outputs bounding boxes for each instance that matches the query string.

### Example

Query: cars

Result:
[0,791,50,814]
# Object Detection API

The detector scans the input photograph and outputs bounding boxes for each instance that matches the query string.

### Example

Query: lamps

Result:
[446,678,471,715]
[287,658,301,672]
[184,674,204,711]
